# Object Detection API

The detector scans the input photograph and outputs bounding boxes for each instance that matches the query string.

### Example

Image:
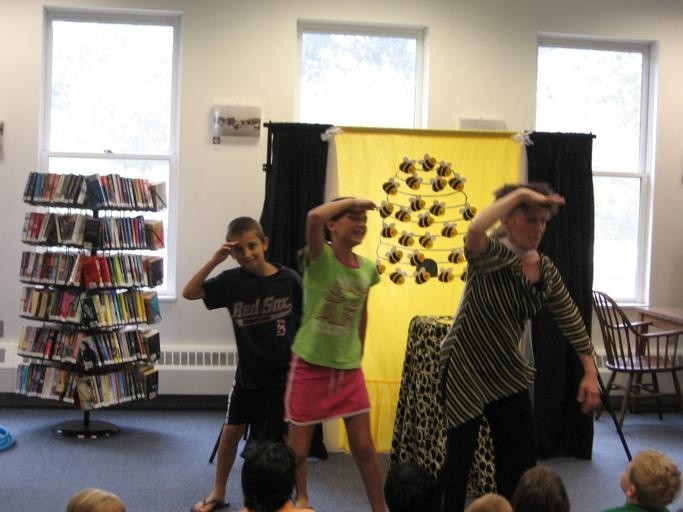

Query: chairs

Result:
[589,290,682,433]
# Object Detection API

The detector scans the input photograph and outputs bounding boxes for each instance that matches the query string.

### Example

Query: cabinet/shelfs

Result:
[14,184,168,439]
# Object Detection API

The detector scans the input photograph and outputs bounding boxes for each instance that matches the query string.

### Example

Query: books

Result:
[12,172,167,413]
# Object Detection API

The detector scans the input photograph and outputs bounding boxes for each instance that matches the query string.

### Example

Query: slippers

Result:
[191,495,230,512]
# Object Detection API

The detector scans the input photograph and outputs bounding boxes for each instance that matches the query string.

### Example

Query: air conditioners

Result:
[151,346,240,396]
[0,342,19,395]
[590,345,682,399]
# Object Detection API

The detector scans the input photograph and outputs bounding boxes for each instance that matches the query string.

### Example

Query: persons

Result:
[383,461,443,510]
[283,195,386,512]
[510,465,571,512]
[180,217,328,511]
[596,449,681,511]
[462,492,510,512]
[235,438,314,512]
[62,486,126,511]
[437,181,601,511]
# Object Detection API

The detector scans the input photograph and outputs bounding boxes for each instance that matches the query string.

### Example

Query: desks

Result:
[630,305,682,420]
[387,315,501,497]
